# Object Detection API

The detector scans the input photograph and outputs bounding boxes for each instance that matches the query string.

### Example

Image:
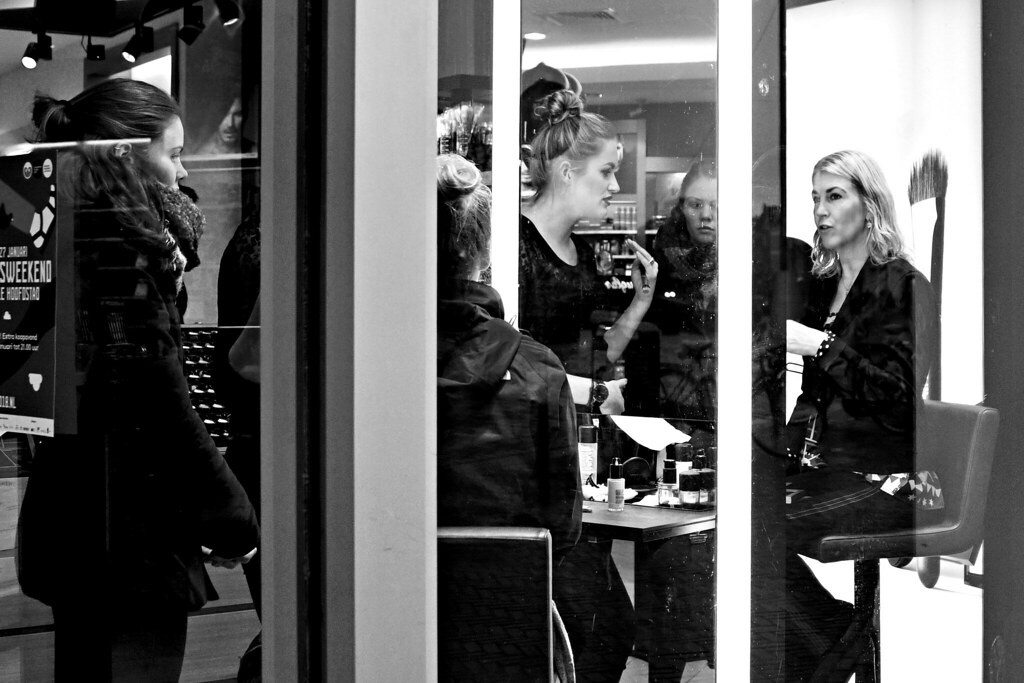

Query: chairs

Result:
[437,524,575,683]
[789,399,1001,683]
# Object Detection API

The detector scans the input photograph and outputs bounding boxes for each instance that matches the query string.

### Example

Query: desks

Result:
[580,500,716,546]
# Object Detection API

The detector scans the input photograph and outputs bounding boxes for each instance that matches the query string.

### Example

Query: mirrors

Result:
[518,0,717,502]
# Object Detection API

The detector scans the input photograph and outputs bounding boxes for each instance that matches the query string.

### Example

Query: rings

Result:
[649,256,655,265]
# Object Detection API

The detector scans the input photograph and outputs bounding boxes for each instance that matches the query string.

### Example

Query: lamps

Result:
[21,0,241,69]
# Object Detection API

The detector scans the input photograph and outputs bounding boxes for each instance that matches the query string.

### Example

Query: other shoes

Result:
[813,609,873,683]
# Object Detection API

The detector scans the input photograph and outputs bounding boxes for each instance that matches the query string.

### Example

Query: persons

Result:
[196,86,258,156]
[13,79,260,683]
[436,87,940,683]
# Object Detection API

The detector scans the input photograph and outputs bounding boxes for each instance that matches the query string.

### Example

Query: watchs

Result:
[588,377,609,414]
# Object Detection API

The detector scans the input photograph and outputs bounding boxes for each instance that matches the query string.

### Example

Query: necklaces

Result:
[841,251,869,296]
